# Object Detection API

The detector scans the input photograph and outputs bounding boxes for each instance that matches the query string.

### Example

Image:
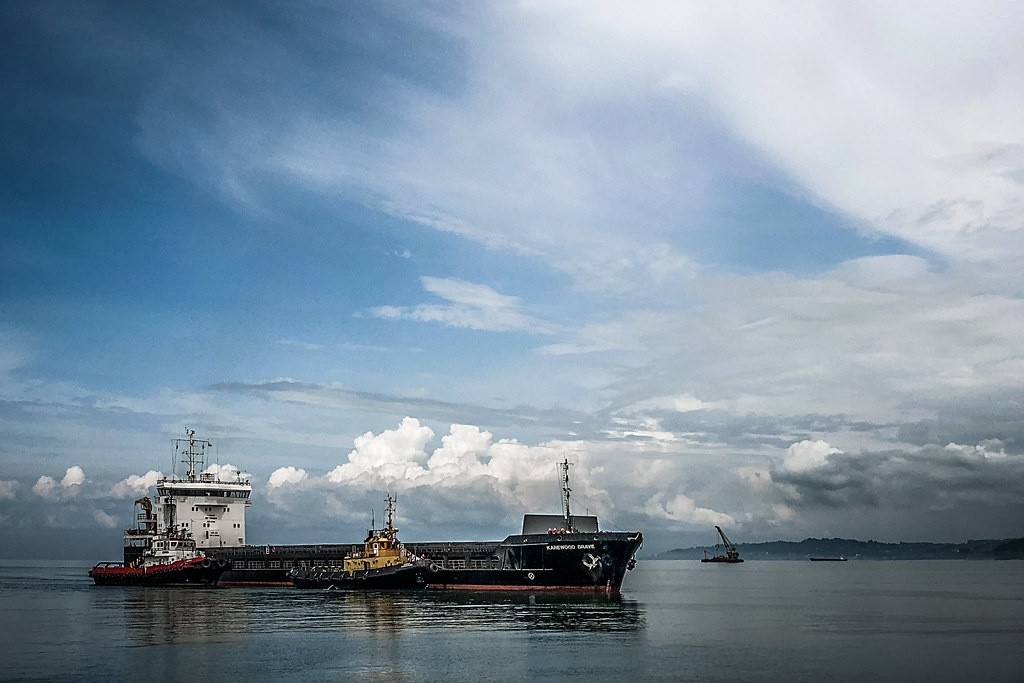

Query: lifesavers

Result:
[553,528,557,534]
[561,528,565,534]
[317,577,322,582]
[351,575,355,580]
[91,566,183,577]
[339,575,343,581]
[430,564,438,574]
[217,558,226,568]
[202,559,212,569]
[328,576,332,582]
[363,574,368,580]
[310,576,315,581]
[548,528,552,534]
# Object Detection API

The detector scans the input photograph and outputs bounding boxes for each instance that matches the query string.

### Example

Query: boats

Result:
[809,557,848,562]
[122,425,644,594]
[87,524,233,589]
[286,491,439,594]
